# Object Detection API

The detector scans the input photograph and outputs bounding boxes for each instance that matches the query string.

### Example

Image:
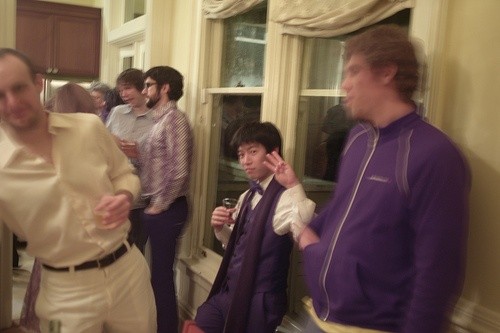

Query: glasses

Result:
[144,82,157,89]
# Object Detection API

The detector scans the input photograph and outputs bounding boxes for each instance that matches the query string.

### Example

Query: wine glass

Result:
[222,198,237,222]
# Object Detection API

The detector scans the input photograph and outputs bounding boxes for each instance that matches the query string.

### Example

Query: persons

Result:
[20,66,194,333]
[194,122,307,333]
[297,25,470,333]
[0,48,158,333]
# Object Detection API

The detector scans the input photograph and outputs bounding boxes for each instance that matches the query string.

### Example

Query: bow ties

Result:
[248,180,264,196]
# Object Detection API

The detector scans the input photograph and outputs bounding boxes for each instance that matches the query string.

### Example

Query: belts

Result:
[41,232,135,272]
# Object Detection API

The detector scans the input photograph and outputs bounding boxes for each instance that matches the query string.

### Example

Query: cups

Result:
[124,137,135,158]
[89,192,115,229]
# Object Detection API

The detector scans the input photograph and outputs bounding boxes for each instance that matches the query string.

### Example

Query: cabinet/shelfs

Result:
[16,0,102,82]
